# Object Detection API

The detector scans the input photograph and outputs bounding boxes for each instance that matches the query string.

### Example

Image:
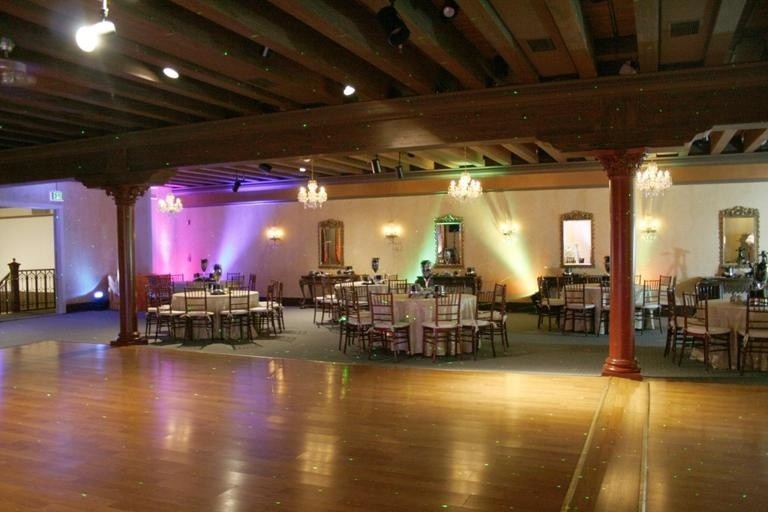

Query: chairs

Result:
[297,268,516,364]
[529,268,679,337]
[108,272,289,345]
[664,280,768,374]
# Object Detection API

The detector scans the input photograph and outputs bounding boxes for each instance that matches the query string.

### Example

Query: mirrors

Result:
[317,217,345,270]
[719,205,760,269]
[433,214,466,269]
[559,209,595,269]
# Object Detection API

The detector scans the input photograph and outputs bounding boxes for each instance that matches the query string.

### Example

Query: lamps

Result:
[158,193,184,216]
[296,155,329,213]
[498,219,518,236]
[743,233,754,262]
[633,161,672,201]
[640,219,663,239]
[381,220,403,239]
[446,143,484,202]
[266,224,286,248]
